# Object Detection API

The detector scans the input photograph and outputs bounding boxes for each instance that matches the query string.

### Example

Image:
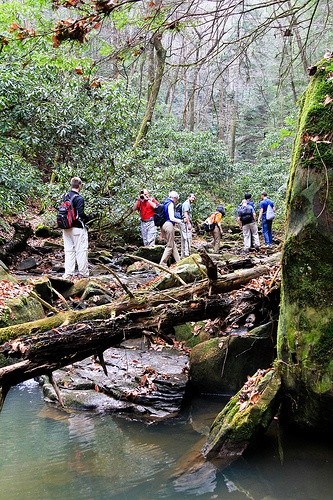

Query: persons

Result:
[209,206,225,254]
[237,199,260,253]
[258,191,276,247]
[244,193,257,213]
[132,189,160,246]
[179,192,197,258]
[159,191,185,269]
[62,177,102,278]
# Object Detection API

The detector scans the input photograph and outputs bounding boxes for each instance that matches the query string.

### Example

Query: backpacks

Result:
[174,199,190,220]
[153,201,171,226]
[57,195,76,228]
[203,212,219,232]
[265,200,275,220]
[240,208,252,221]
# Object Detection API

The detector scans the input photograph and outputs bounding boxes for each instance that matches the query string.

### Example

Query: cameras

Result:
[140,190,144,199]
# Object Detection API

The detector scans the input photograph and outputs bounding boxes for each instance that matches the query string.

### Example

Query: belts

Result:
[142,219,154,222]
